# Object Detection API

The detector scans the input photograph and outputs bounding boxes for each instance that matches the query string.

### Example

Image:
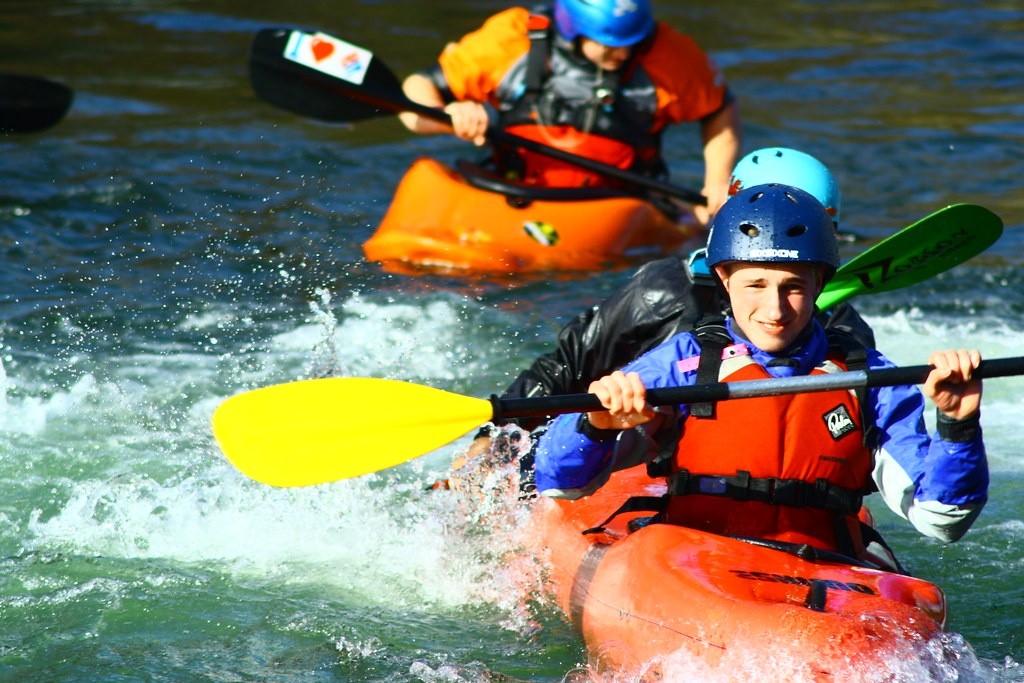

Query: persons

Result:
[537,182,989,544]
[468,147,877,460]
[398,0,741,214]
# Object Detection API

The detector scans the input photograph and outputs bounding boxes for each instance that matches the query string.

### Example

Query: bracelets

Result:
[574,414,623,443]
[938,412,979,438]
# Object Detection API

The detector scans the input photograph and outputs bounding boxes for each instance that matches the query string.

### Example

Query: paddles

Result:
[424,203,1005,495]
[211,356,1024,486]
[246,27,709,208]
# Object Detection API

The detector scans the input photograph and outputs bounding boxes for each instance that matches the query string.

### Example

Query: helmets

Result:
[727,147,841,222]
[555,0,653,47]
[705,182,841,267]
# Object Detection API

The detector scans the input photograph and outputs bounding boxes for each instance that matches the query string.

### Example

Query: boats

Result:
[446,436,948,682]
[359,158,704,286]
